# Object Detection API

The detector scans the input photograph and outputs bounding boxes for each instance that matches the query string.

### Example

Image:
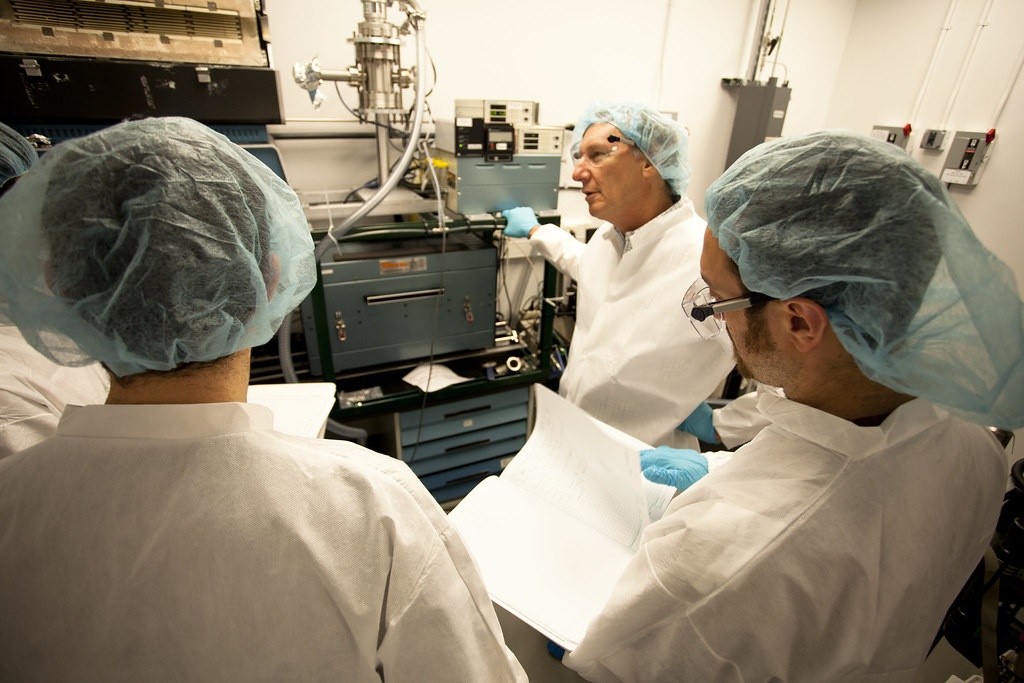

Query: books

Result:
[247,383,337,437]
[448,385,677,649]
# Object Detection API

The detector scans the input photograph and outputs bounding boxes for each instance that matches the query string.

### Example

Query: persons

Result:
[0,122,111,460]
[0,116,528,683]
[502,105,787,660]
[562,134,1009,683]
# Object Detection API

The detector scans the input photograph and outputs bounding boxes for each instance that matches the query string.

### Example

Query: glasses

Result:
[570,135,636,168]
[682,276,780,340]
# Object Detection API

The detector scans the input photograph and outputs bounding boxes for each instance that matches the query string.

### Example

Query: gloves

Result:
[640,446,708,490]
[676,401,719,443]
[501,206,539,238]
